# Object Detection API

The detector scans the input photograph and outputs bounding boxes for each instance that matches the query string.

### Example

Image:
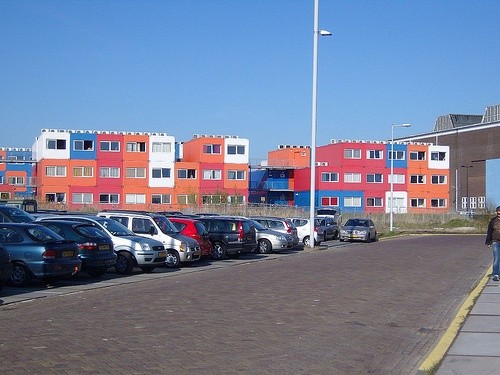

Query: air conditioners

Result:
[0,147,31,151]
[322,162,328,166]
[330,139,433,146]
[315,162,320,166]
[278,144,310,149]
[41,129,166,136]
[194,134,238,138]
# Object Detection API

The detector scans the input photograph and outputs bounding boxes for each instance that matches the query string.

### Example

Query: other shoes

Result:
[492,274,499,281]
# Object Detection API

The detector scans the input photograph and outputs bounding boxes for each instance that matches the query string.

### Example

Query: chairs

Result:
[138,221,143,231]
[133,222,137,230]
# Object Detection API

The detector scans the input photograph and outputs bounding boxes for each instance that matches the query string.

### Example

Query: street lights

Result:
[309,29,332,248]
[389,124,411,231]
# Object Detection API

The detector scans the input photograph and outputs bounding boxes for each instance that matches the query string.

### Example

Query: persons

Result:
[484,206,500,281]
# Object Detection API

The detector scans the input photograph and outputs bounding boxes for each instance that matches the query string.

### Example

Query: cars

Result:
[338,217,376,244]
[0,222,83,289]
[0,201,340,283]
